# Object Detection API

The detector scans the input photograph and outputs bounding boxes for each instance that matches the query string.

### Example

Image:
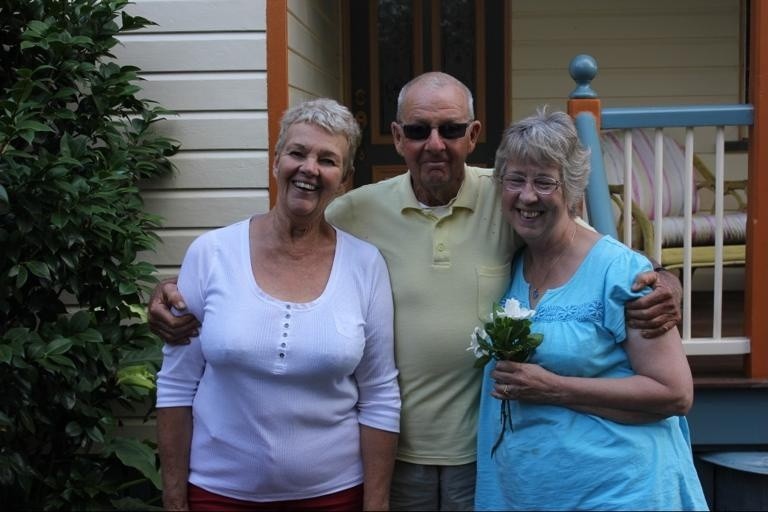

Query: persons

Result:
[147,70,685,511]
[471,103,711,512]
[158,97,403,511]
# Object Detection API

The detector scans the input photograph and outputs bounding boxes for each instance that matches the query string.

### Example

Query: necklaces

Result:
[527,225,578,305]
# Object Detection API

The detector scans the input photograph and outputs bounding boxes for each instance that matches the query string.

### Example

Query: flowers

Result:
[465,297,543,460]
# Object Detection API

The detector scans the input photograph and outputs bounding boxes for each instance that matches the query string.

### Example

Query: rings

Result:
[503,383,513,397]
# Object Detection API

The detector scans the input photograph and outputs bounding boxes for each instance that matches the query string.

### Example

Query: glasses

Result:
[500,174,563,195]
[401,123,469,139]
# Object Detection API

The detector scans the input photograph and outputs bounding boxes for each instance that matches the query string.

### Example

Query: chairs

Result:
[562,125,749,288]
